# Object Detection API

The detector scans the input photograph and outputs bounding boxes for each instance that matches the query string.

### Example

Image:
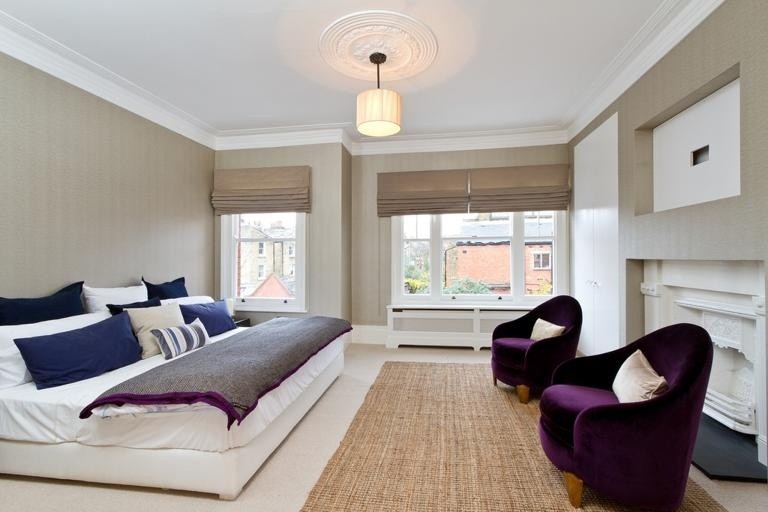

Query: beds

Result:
[0,315,353,499]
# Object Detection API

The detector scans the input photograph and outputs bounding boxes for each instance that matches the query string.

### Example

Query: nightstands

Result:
[234,316,251,325]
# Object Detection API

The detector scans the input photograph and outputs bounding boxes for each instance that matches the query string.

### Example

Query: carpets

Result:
[298,360,729,512]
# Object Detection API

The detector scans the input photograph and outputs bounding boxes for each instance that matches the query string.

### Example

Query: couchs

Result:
[538,322,713,511]
[491,294,583,404]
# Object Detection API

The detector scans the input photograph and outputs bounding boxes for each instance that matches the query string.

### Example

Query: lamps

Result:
[355,54,402,138]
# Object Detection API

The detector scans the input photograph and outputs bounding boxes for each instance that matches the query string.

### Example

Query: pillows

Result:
[122,303,186,359]
[150,317,211,362]
[13,310,144,389]
[106,295,160,343]
[530,318,566,342]
[81,281,149,312]
[160,295,214,322]
[611,349,667,406]
[0,307,111,389]
[142,276,188,301]
[179,300,236,338]
[0,280,85,324]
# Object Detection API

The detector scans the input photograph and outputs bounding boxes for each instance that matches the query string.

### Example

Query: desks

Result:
[384,304,475,351]
[475,304,538,351]
